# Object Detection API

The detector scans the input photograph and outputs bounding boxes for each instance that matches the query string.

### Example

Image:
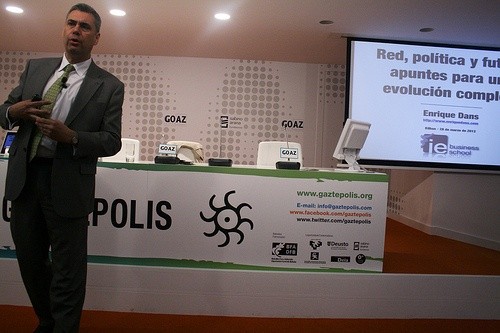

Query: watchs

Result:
[72,132,79,145]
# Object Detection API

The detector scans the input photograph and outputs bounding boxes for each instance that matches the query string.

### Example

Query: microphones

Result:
[61,77,67,83]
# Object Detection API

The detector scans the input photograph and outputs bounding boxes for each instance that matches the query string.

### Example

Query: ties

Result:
[27,64,75,163]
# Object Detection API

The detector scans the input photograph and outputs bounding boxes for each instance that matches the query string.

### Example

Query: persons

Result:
[0,3,126,333]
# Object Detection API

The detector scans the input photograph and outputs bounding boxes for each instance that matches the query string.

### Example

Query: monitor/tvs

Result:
[333,118,371,173]
[2,131,16,154]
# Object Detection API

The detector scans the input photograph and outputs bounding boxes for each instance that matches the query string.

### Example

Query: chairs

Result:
[102,138,140,162]
[257,141,302,168]
[163,141,203,163]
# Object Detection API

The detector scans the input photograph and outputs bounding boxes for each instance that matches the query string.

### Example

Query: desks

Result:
[0,155,389,274]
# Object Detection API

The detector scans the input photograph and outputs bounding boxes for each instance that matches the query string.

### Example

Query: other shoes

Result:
[33,326,54,333]
